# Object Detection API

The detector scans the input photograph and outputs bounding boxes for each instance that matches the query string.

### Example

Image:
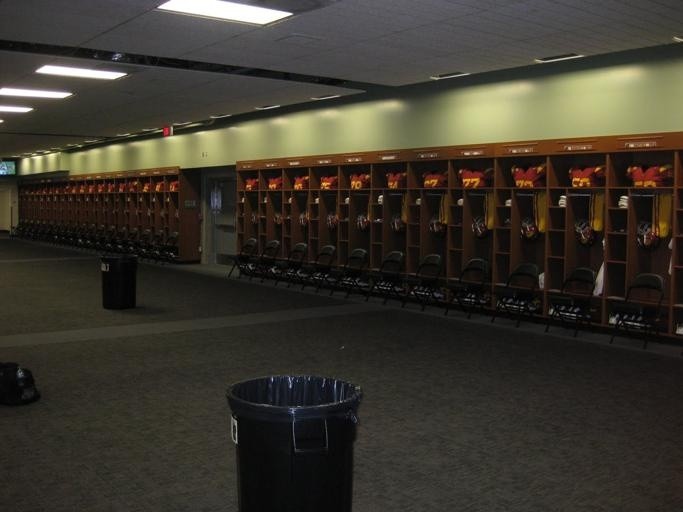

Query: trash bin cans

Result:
[226,375,362,512]
[102,254,138,310]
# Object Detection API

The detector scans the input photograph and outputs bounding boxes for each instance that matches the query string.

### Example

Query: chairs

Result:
[14,221,180,265]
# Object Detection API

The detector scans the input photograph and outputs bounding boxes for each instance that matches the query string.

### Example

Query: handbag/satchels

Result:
[0,362,40,406]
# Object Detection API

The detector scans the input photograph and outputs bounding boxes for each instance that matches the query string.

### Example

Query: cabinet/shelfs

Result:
[236,131,683,344]
[19,166,201,264]
